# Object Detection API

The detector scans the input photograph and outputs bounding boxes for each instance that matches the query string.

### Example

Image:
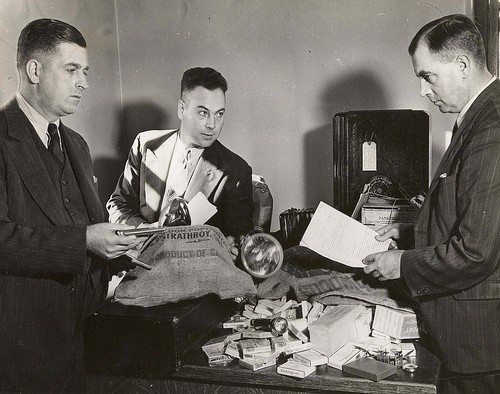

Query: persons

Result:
[362,14,500,394]
[0,18,152,394]
[106,67,252,265]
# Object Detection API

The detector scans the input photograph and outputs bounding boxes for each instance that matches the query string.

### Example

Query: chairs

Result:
[279,208,316,250]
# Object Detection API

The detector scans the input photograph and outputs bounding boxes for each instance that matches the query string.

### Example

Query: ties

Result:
[46,123,65,176]
[451,121,458,140]
[171,149,188,197]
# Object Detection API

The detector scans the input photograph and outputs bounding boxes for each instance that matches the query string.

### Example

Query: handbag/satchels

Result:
[111,225,258,308]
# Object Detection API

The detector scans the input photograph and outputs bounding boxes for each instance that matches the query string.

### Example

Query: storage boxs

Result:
[85,293,227,374]
